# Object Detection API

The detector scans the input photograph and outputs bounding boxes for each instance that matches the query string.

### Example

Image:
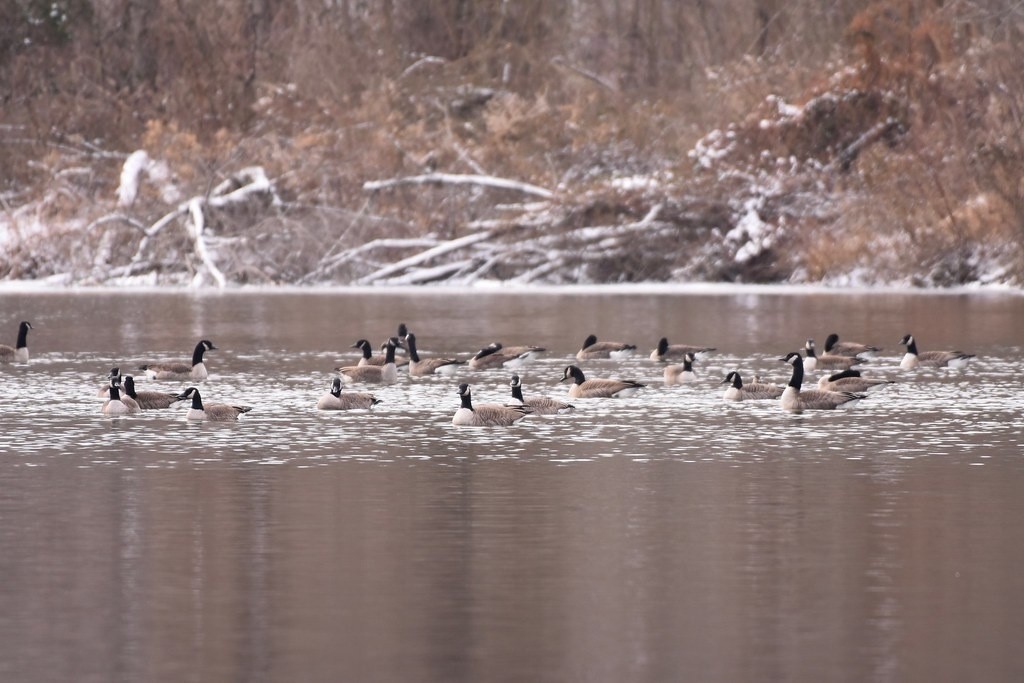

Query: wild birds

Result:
[0,321,35,364]
[96,339,253,422]
[316,323,977,428]
